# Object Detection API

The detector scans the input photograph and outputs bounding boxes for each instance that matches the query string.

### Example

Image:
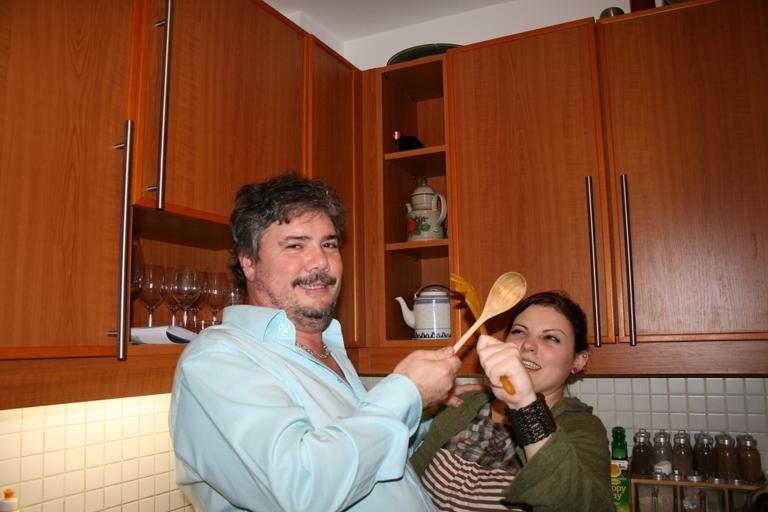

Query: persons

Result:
[171,170,491,512]
[410,292,617,512]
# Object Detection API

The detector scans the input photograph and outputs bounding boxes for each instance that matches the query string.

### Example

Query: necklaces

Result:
[294,339,333,358]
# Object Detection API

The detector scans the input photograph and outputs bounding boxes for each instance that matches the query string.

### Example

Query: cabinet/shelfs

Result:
[310,33,368,348]
[368,54,455,378]
[443,1,767,379]
[127,1,308,251]
[1,2,138,413]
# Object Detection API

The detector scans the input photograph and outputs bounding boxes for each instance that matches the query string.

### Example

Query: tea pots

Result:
[394,282,453,341]
[402,177,446,242]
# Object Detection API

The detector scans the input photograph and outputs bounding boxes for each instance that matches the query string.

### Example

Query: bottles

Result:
[609,427,632,511]
[630,421,762,483]
[391,131,425,151]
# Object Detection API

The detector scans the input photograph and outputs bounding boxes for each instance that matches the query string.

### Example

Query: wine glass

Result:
[130,261,244,332]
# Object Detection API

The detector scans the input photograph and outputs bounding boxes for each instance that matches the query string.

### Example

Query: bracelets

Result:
[504,393,557,447]
